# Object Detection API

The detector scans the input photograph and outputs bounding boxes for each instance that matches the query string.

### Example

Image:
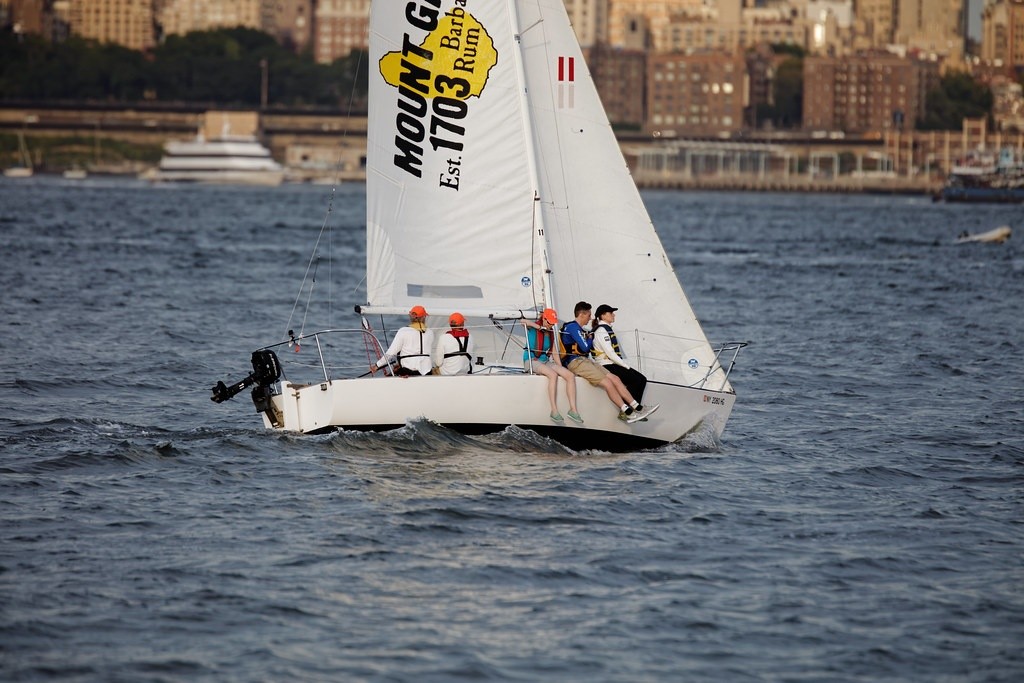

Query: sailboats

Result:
[209,1,747,446]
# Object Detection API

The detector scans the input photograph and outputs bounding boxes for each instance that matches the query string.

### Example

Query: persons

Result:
[433,314,475,376]
[520,301,660,423]
[371,307,436,378]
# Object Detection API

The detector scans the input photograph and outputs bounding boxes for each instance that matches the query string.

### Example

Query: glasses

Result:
[544,317,555,326]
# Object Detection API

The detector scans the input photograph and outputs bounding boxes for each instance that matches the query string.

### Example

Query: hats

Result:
[595,304,618,317]
[449,313,466,326]
[543,309,558,324]
[408,306,429,317]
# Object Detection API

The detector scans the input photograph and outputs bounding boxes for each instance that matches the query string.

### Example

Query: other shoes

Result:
[567,410,584,423]
[626,409,650,423]
[550,412,564,422]
[618,410,648,422]
[634,403,660,418]
